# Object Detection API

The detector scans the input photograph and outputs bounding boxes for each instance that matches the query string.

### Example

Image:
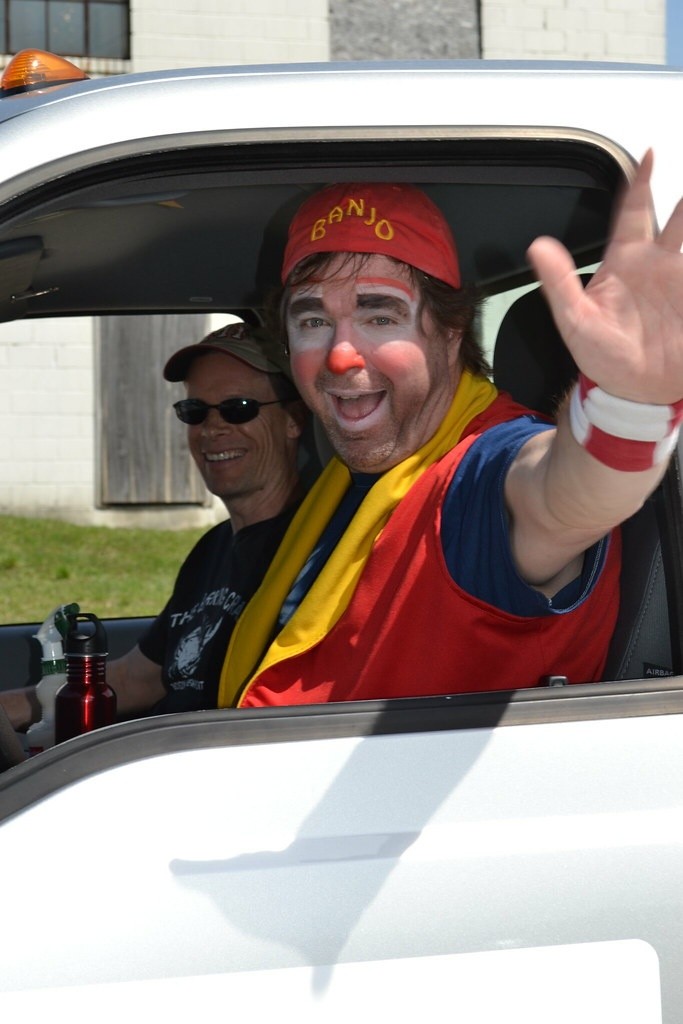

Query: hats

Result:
[281,182,462,291]
[163,322,295,388]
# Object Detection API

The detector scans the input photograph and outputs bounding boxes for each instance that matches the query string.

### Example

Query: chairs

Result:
[492,273,675,684]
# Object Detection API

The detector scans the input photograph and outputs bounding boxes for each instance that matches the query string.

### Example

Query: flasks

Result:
[55,613,117,745]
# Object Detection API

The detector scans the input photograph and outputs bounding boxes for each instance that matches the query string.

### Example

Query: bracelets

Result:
[568,375,682,474]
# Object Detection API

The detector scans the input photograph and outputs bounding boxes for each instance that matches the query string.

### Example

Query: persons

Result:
[0,321,324,774]
[201,145,683,714]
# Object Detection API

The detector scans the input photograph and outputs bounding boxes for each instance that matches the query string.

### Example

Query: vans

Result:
[0,49,683,1024]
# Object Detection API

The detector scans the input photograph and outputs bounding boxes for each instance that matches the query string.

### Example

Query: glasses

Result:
[173,397,296,425]
